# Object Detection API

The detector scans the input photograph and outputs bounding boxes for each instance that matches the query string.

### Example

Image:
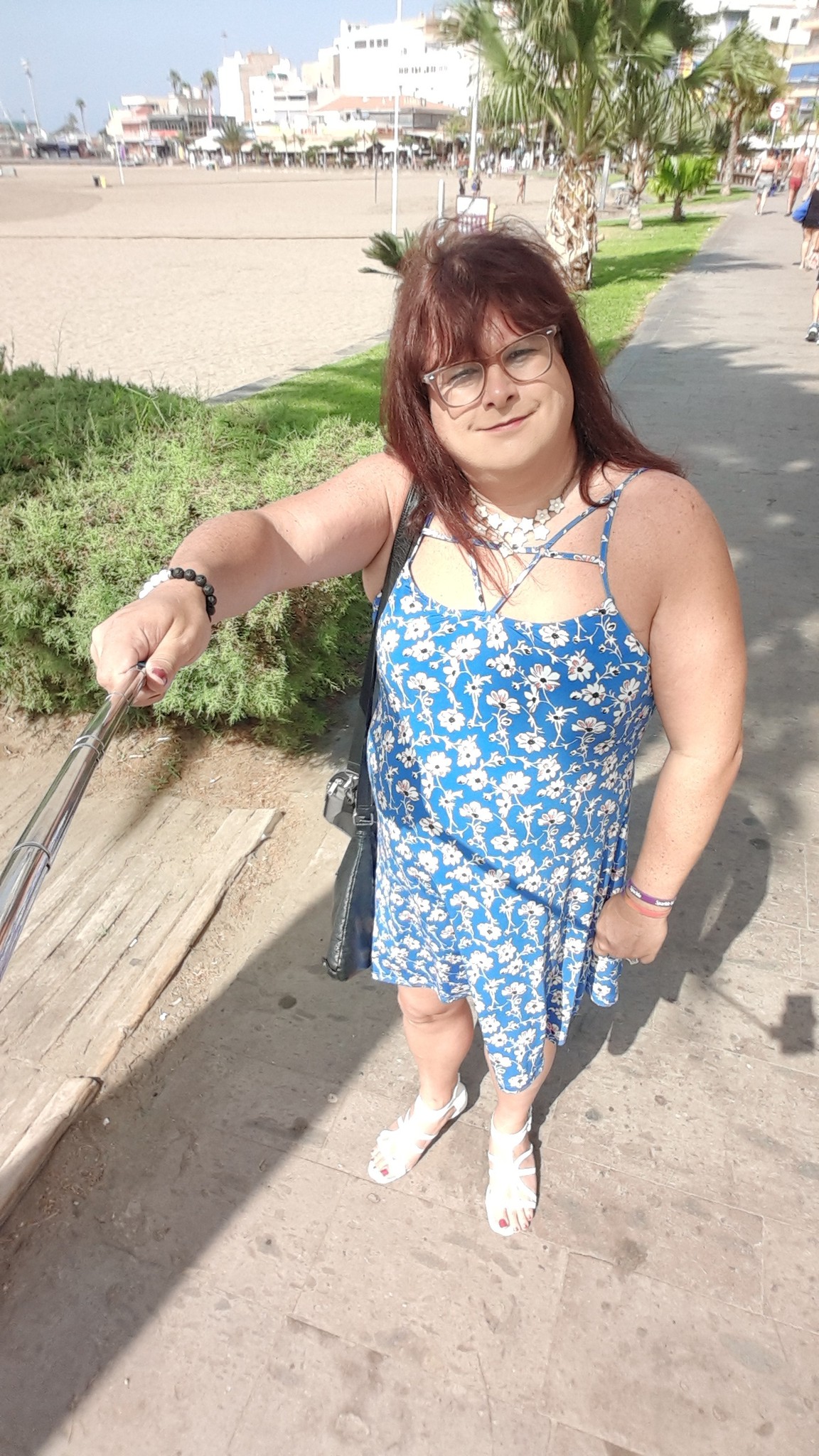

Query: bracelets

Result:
[136,567,216,624]
[627,878,676,907]
[621,885,672,918]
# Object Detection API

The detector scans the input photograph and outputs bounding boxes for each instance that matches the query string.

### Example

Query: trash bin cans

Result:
[455,196,489,234]
[92,175,106,189]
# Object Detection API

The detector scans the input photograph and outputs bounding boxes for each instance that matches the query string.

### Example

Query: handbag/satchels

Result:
[326,821,374,981]
[791,196,811,223]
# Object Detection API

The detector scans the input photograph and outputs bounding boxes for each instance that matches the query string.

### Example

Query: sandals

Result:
[485,1106,538,1236]
[369,1073,468,1184]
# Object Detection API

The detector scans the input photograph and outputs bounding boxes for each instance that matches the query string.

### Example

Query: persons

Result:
[93,210,747,1236]
[459,176,528,203]
[751,145,819,344]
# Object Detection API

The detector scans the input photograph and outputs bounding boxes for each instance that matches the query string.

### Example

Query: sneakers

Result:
[805,323,819,342]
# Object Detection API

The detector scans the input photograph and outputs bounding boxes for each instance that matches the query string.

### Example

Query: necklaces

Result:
[464,488,566,559]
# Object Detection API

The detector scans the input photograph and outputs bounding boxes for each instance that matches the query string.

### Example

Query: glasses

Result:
[420,324,557,408]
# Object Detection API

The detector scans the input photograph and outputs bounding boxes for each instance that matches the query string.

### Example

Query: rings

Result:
[625,958,639,966]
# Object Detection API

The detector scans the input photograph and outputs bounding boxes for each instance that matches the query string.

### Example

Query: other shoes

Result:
[784,212,792,217]
